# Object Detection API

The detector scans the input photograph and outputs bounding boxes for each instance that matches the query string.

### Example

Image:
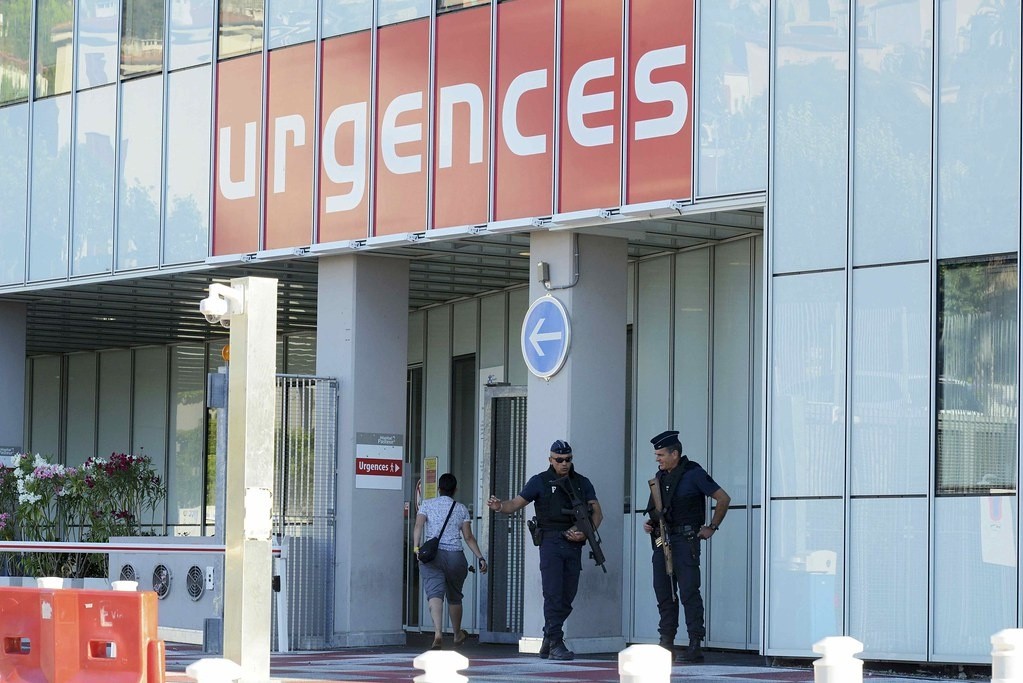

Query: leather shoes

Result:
[547,639,575,659]
[538,636,550,658]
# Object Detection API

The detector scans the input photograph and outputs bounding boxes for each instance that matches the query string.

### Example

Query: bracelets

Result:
[413,546,419,554]
[479,557,485,563]
[495,502,504,513]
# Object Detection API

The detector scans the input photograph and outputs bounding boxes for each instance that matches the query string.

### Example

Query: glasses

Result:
[549,455,572,463]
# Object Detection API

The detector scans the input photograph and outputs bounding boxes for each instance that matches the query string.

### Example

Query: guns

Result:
[681,529,699,562]
[647,477,677,602]
[555,475,608,574]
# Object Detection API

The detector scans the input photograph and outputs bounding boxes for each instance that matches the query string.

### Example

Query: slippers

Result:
[453,629,467,647]
[430,644,441,650]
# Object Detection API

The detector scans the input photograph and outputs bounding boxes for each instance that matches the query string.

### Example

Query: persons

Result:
[487,439,604,661]
[643,430,731,663]
[413,473,487,651]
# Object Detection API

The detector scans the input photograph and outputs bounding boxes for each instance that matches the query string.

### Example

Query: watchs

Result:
[709,524,719,531]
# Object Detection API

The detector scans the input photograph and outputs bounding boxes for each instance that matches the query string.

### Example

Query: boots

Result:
[658,633,675,661]
[674,636,705,663]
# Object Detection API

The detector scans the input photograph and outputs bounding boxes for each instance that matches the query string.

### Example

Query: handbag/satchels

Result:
[417,536,438,564]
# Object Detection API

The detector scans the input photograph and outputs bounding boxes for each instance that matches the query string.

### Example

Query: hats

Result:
[549,439,572,454]
[649,430,680,449]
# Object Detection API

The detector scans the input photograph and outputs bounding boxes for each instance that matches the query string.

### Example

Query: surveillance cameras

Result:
[200,297,231,328]
